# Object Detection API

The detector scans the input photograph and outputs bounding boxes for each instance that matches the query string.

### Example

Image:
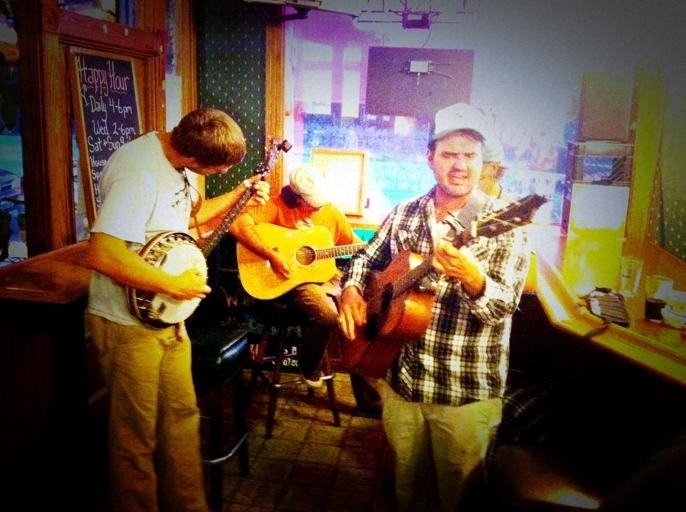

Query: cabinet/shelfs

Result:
[0,239,147,506]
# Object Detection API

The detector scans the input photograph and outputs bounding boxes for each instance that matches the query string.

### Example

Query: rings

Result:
[243,178,252,187]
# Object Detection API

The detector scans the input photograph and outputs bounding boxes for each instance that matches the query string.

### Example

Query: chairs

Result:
[560,72,647,234]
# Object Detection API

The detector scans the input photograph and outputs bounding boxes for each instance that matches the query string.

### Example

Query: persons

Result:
[333,102,534,511]
[477,134,514,203]
[228,160,371,388]
[81,106,270,511]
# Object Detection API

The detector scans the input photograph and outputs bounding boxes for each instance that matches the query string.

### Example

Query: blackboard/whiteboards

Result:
[68,45,143,231]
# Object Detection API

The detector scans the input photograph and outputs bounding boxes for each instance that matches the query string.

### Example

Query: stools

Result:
[246,290,349,442]
[461,379,540,486]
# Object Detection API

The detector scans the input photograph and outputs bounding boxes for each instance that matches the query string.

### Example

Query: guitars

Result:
[339,192,555,379]
[237,221,367,302]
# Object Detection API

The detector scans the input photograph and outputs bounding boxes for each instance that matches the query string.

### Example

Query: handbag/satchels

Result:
[588,296,628,327]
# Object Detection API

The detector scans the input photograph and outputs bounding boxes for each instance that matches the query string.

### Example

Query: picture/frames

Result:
[66,43,160,251]
[305,146,367,216]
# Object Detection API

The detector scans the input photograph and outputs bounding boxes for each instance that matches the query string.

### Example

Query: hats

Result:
[289,164,334,208]
[482,133,505,161]
[429,103,488,146]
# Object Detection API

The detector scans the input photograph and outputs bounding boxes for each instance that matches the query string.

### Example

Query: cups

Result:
[618,255,644,297]
[641,274,673,323]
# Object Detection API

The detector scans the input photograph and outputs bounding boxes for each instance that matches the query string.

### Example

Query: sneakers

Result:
[302,361,323,388]
